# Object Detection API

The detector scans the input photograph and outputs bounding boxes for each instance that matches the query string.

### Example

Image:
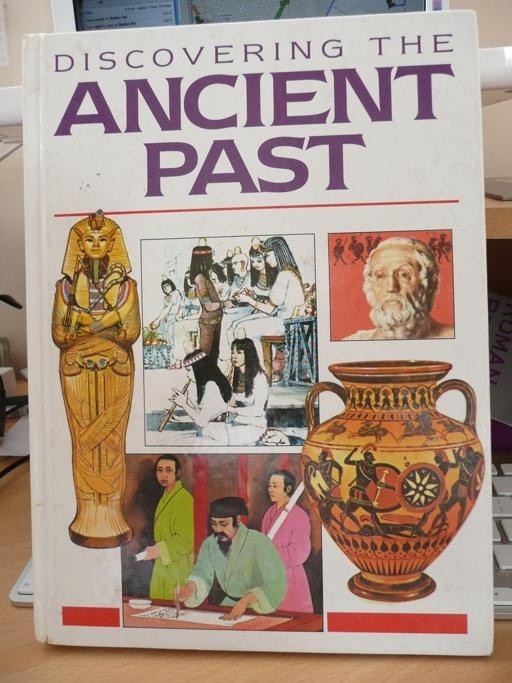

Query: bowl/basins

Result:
[127,597,154,611]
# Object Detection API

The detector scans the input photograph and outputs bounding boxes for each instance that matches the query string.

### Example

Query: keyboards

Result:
[8,462,511,620]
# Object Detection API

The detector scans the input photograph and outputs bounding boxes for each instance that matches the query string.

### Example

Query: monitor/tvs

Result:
[51,0,448,35]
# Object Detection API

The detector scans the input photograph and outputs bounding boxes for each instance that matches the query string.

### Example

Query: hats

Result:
[210,496,248,518]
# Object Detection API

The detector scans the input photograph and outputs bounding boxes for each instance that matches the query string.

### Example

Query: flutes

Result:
[157,379,192,432]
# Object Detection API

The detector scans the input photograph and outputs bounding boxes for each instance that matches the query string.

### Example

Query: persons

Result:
[144,457,313,621]
[151,236,305,446]
[340,236,455,340]
[51,210,141,539]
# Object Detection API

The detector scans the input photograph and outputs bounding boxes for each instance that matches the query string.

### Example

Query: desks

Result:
[0,413,511,682]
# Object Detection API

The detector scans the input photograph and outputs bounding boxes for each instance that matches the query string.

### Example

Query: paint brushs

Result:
[176,575,180,619]
